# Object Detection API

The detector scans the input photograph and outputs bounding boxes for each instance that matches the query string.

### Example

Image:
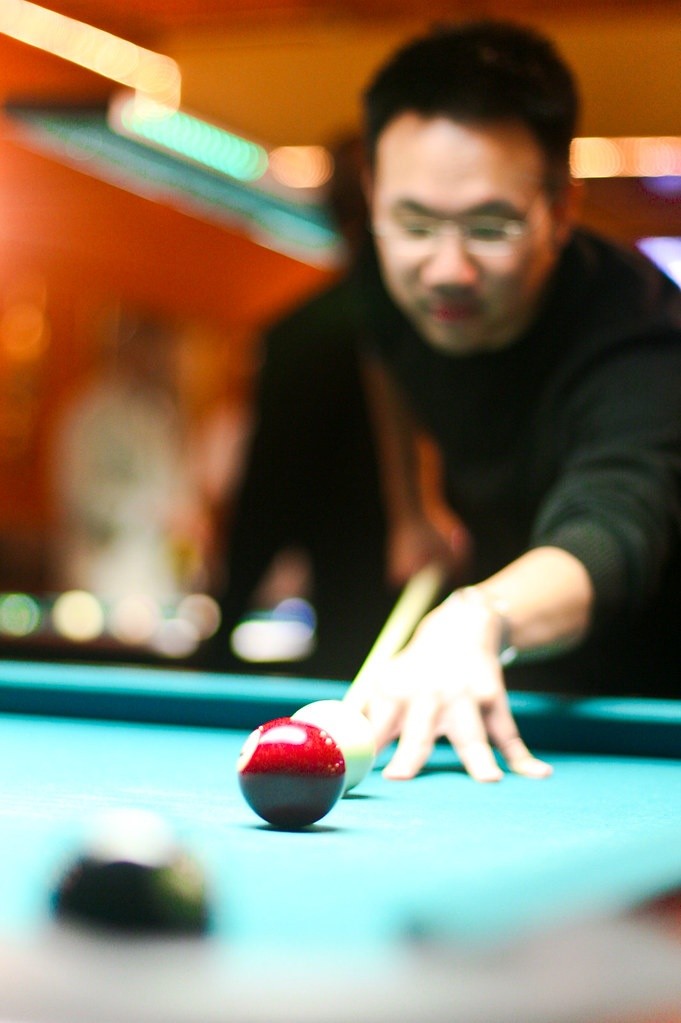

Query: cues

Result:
[340,562,449,712]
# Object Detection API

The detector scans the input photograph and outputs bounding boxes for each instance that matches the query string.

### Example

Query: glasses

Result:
[371,184,548,253]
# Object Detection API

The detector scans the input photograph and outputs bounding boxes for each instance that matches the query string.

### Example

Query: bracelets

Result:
[459,585,518,668]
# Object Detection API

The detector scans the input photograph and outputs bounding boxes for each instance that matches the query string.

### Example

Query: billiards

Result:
[236,698,378,829]
[50,826,216,937]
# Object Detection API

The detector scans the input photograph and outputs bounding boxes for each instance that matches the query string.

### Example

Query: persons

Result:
[215,18,681,784]
[47,323,206,622]
